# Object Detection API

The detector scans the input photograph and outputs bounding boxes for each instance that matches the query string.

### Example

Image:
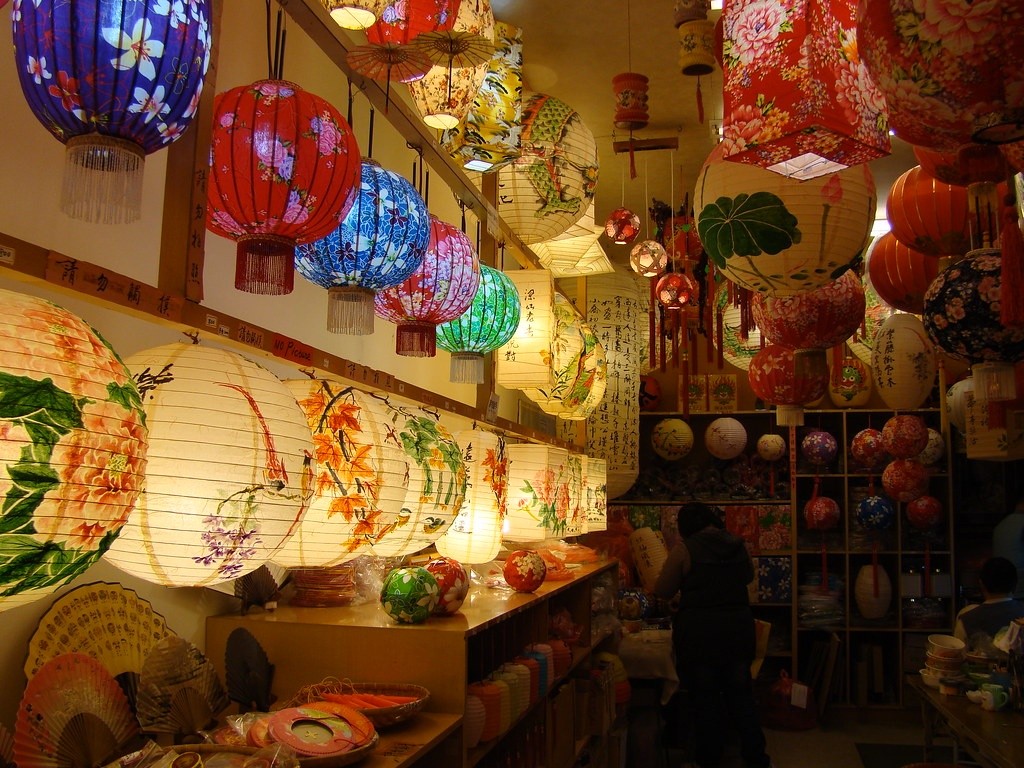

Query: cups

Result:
[925,662,961,677]
[925,651,964,670]
[982,683,1008,712]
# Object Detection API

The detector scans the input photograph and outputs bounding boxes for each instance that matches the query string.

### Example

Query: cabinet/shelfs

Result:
[156,408,1024,768]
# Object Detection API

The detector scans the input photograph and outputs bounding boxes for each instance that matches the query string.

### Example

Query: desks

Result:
[907,675,1024,768]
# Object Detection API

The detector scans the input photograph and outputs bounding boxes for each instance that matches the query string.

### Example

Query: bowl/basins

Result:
[926,650,963,660]
[919,668,965,688]
[966,651,1007,664]
[927,633,965,656]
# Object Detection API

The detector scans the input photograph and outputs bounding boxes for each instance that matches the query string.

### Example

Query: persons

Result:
[653,500,772,768]
[953,556,1024,653]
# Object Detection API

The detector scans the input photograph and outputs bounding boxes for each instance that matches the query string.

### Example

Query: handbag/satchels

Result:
[671,610,737,663]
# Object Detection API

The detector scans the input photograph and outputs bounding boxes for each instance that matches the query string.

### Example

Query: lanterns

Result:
[0,0,1024,626]
